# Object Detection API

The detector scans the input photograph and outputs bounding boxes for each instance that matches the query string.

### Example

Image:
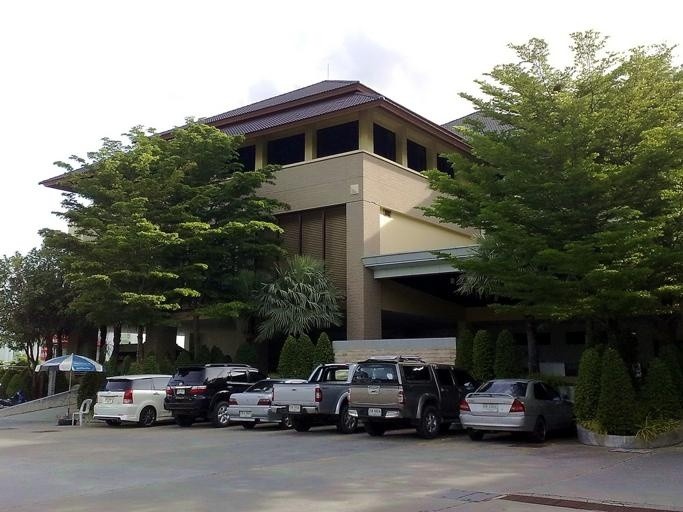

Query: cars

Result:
[227,377,307,429]
[458,378,574,443]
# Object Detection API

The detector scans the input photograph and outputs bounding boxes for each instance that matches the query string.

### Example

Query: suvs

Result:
[347,355,477,439]
[163,361,267,428]
[91,373,173,425]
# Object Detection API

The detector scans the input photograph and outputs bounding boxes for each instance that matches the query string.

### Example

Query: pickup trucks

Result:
[271,363,357,434]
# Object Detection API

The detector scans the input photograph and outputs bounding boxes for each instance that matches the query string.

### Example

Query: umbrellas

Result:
[34,352,107,418]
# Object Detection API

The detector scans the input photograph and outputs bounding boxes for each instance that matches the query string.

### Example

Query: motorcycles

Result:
[0,390,27,409]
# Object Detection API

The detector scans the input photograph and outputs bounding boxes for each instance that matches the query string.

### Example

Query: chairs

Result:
[72,399,93,426]
